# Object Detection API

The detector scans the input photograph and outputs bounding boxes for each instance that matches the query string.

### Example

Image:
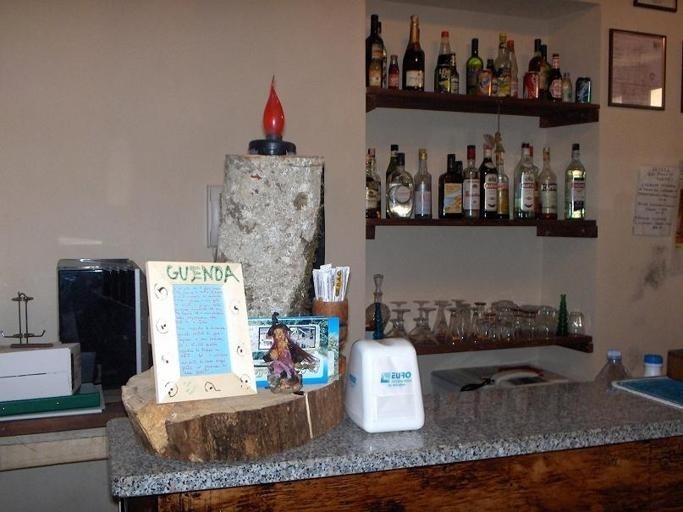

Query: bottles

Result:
[642,354,664,376]
[593,350,634,381]
[364,274,392,332]
[364,14,571,101]
[366,140,587,219]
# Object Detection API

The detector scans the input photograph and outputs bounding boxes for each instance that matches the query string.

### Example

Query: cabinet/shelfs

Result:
[366,88,599,356]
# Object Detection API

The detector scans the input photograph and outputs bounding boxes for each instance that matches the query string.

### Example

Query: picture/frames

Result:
[608,28,666,111]
[632,0,678,12]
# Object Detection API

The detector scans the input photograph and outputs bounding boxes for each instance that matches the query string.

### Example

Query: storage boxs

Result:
[0,342,82,402]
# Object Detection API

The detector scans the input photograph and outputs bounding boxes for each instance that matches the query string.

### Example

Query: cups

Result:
[311,297,348,348]
[488,298,585,344]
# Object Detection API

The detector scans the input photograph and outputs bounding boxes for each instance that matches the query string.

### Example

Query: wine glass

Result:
[389,298,489,346]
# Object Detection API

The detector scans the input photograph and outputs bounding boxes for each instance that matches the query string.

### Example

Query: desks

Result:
[157,433,683,512]
[0,384,130,512]
